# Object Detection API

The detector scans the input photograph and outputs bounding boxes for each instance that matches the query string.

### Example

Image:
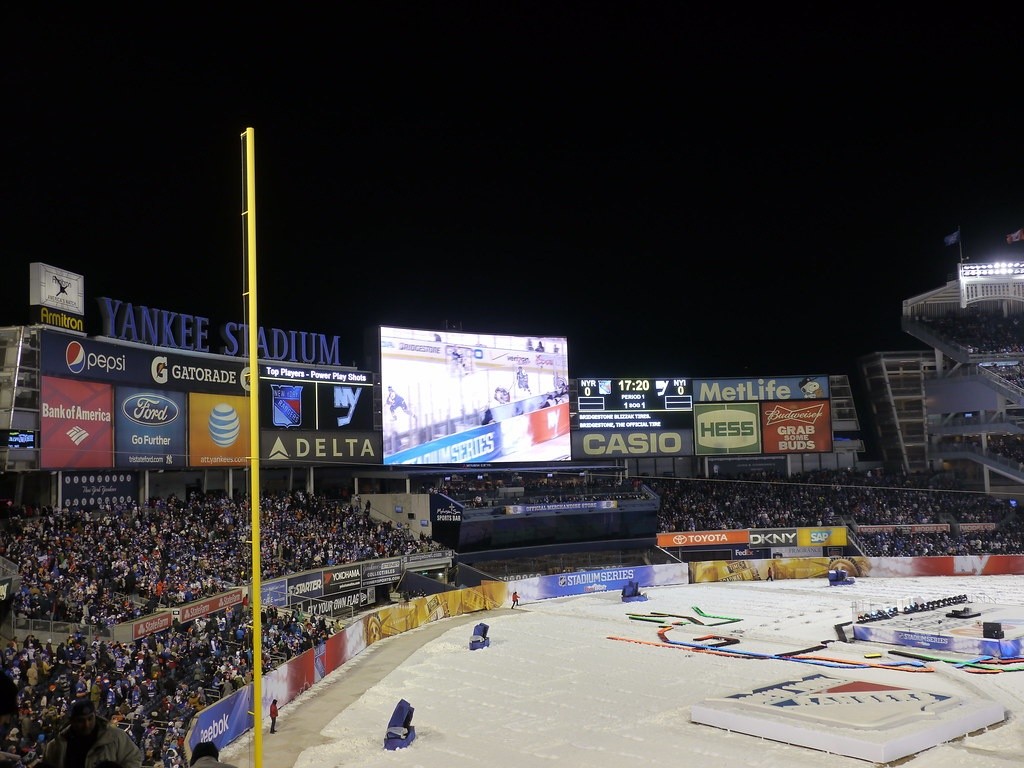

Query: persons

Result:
[0,492,453,768]
[511,591,520,608]
[638,463,1024,560]
[424,463,654,523]
[383,334,570,454]
[765,566,773,581]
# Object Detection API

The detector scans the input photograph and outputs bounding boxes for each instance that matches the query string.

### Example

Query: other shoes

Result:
[387,417,398,422]
[766,579,768,581]
[771,580,773,581]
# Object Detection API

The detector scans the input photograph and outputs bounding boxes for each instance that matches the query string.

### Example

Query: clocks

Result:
[29,262,84,317]
